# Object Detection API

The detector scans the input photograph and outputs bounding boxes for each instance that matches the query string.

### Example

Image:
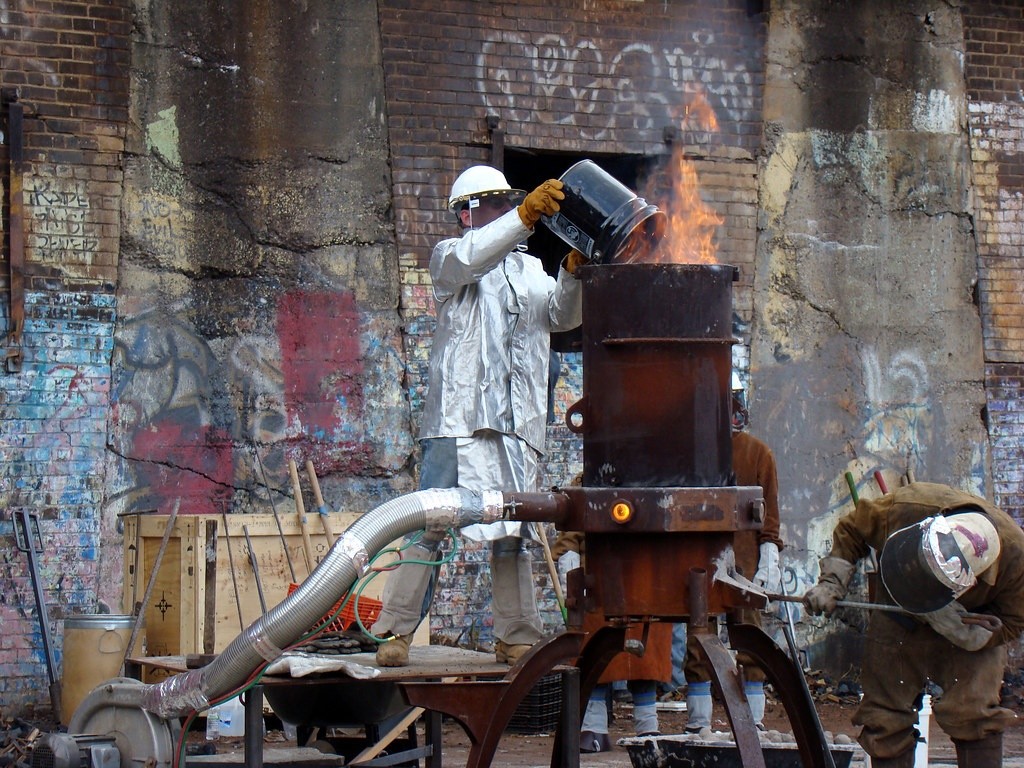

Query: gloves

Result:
[519,179,564,229]
[803,557,857,619]
[567,249,589,273]
[753,542,781,611]
[559,551,581,600]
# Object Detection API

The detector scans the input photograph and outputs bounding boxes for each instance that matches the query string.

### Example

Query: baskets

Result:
[476,675,561,735]
[289,583,382,634]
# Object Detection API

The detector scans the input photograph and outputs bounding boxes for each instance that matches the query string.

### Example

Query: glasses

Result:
[488,196,512,209]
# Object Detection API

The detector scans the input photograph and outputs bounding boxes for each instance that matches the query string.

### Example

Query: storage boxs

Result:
[125,512,429,718]
[477,673,564,734]
[287,584,383,635]
[615,732,853,768]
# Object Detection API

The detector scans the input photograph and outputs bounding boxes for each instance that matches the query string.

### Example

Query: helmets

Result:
[448,165,527,214]
[924,601,992,652]
[880,514,1000,614]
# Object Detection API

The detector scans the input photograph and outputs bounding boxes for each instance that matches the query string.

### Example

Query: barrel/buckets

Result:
[537,158,670,265]
[578,258,742,487]
[58,612,148,730]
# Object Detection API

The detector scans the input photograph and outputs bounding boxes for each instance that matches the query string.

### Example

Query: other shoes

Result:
[376,634,414,665]
[495,642,532,664]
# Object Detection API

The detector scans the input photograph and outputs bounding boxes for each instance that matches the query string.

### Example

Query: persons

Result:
[548,470,673,754]
[371,166,588,667]
[680,389,784,737]
[802,481,1022,767]
